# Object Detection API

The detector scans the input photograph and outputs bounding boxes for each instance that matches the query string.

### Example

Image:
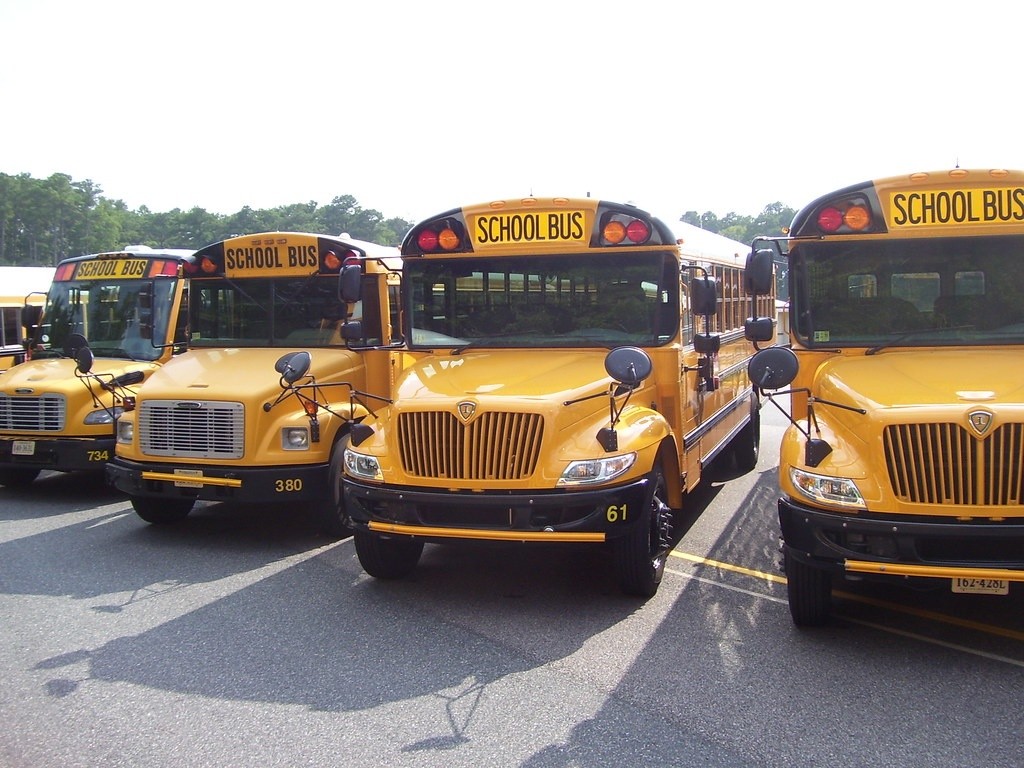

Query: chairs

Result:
[808,294,1024,335]
[455,282,669,336]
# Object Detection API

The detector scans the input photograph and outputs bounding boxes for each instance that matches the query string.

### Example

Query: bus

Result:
[0,245,198,496]
[744,168,1024,635]
[274,193,778,596]
[76,230,670,539]
[0,264,60,375]
